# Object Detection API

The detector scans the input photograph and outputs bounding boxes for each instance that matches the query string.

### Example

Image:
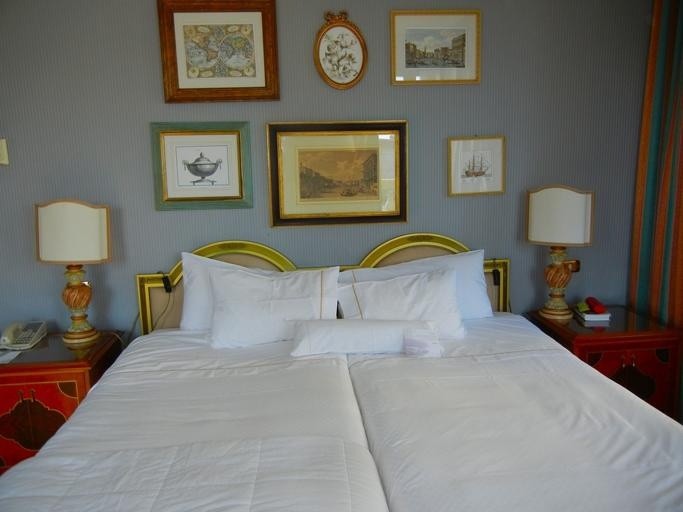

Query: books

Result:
[575,313,610,327]
[575,304,611,321]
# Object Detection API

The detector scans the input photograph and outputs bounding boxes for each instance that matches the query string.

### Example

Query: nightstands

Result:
[528,301,679,424]
[0,330,122,477]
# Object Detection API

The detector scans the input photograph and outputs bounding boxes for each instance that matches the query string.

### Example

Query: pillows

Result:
[179,251,341,336]
[290,314,444,360]
[336,250,494,324]
[208,268,341,351]
[335,270,467,341]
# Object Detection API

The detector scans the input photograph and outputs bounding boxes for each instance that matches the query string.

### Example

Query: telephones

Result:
[0,320,48,350]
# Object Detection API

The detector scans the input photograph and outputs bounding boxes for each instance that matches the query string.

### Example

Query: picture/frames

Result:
[266,120,411,227]
[148,121,256,211]
[314,9,369,91]
[388,7,483,85]
[444,135,507,198]
[155,0,284,104]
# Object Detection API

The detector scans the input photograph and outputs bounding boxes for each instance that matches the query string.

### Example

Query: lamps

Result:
[32,199,113,345]
[523,185,595,318]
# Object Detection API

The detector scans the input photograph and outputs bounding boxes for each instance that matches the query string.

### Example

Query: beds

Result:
[1,231,683,511]
